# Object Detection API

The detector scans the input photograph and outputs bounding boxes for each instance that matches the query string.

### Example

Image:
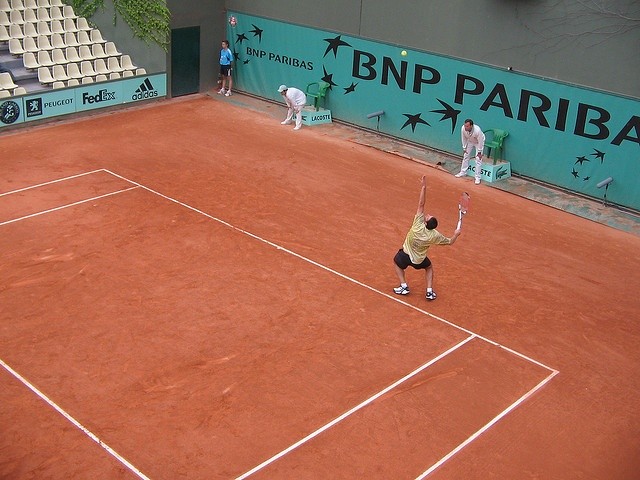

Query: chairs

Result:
[9,22,25,39]
[37,51,56,67]
[23,0,38,9]
[0,11,11,26]
[0,90,12,99]
[64,32,80,47]
[51,20,66,34]
[120,55,137,71]
[10,0,25,10]
[50,6,64,20]
[23,36,41,53]
[64,18,79,32]
[37,0,51,8]
[9,38,28,56]
[94,59,111,74]
[52,65,71,82]
[63,6,79,19]
[0,0,11,12]
[79,46,97,61]
[53,81,66,90]
[105,42,122,56]
[107,57,125,73]
[68,79,79,87]
[66,63,85,79]
[10,9,25,24]
[77,31,94,46]
[37,35,55,50]
[52,49,70,65]
[50,34,68,48]
[22,21,39,37]
[37,6,51,21]
[122,71,134,79]
[303,80,330,113]
[109,73,120,80]
[135,68,147,76]
[14,87,27,96]
[50,0,64,7]
[479,128,510,164]
[90,29,107,44]
[92,44,109,59]
[65,46,82,63]
[0,72,19,90]
[23,52,42,69]
[24,7,38,23]
[96,75,107,83]
[37,21,52,36]
[37,67,56,85]
[0,25,11,41]
[77,18,93,30]
[82,77,94,84]
[81,61,97,77]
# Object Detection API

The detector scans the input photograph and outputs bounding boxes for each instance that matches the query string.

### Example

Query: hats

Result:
[277,85,286,92]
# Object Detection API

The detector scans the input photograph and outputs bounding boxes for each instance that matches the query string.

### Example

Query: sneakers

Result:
[455,171,467,178]
[426,292,436,300]
[393,283,410,295]
[224,91,231,96]
[475,177,481,185]
[217,89,226,95]
[293,126,300,130]
[281,119,291,125]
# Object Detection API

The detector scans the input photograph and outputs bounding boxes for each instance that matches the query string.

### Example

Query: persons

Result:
[393,175,461,300]
[216,40,234,96]
[454,119,485,185]
[277,85,307,130]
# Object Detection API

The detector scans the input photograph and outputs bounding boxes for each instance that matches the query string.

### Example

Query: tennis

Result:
[401,51,407,55]
